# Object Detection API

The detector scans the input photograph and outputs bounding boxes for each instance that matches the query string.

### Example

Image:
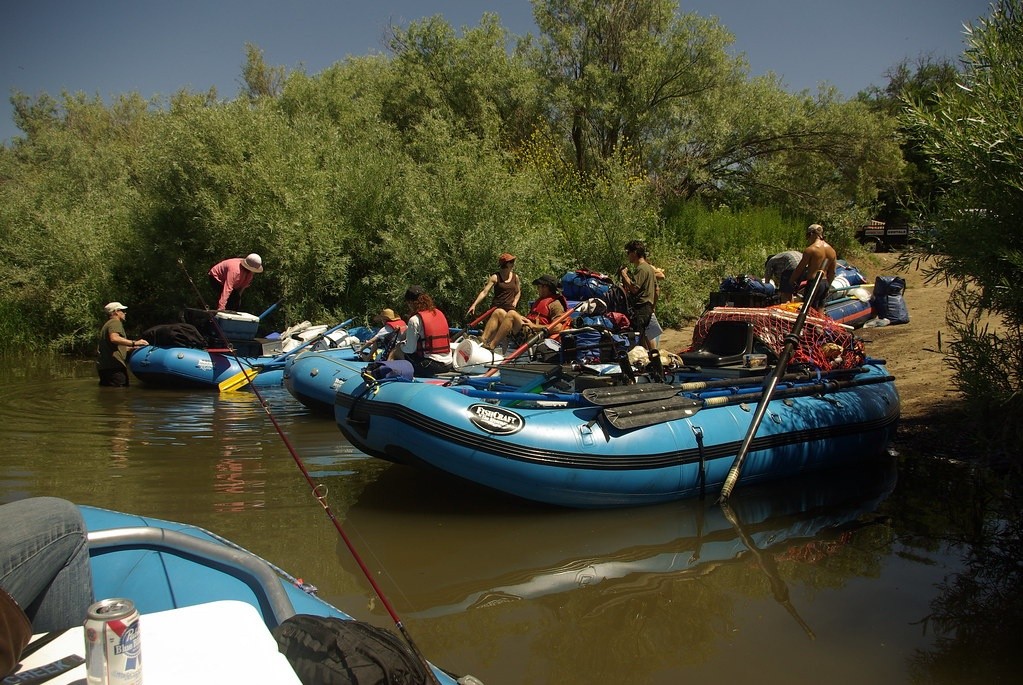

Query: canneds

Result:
[83,598,144,685]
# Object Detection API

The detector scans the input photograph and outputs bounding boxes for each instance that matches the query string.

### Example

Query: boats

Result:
[283,316,659,414]
[333,361,899,508]
[0,504,483,685]
[691,285,877,352]
[129,326,390,391]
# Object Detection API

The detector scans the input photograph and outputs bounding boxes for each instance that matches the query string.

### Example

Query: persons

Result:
[765,223,837,316]
[464,253,521,355]
[621,241,665,349]
[353,308,408,362]
[387,286,453,377]
[463,274,568,353]
[0,496,95,681]
[96,302,149,388]
[208,253,263,311]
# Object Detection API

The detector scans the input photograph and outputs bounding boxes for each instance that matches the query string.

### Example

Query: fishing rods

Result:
[451,340,627,371]
[582,171,621,268]
[173,252,442,684]
[525,143,620,356]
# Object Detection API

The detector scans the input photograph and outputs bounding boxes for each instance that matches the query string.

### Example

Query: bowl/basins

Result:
[742,353,766,367]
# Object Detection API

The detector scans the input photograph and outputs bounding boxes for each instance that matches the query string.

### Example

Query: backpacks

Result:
[873,274,910,324]
[143,322,207,348]
[276,613,432,684]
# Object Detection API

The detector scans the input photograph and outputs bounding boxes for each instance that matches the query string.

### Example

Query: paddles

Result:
[483,301,584,377]
[217,311,359,394]
[719,503,819,642]
[452,303,500,340]
[259,298,283,320]
[714,255,830,506]
[207,348,237,353]
[252,349,382,367]
[581,365,897,432]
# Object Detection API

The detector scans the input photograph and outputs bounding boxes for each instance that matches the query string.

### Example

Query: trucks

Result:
[856,222,908,253]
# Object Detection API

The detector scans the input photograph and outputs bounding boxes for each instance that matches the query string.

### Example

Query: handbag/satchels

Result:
[559,325,640,362]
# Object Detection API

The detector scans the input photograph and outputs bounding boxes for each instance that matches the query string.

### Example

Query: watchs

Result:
[132,340,135,347]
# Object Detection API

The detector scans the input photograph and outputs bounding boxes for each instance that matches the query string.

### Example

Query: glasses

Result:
[627,250,632,255]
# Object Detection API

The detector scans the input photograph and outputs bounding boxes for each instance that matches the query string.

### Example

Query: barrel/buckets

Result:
[826,277,850,299]
[324,330,351,349]
[452,339,504,375]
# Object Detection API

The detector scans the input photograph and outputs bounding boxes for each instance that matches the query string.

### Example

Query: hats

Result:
[104,302,128,314]
[241,253,264,273]
[531,274,559,291]
[403,285,423,303]
[374,308,401,321]
[497,253,516,269]
